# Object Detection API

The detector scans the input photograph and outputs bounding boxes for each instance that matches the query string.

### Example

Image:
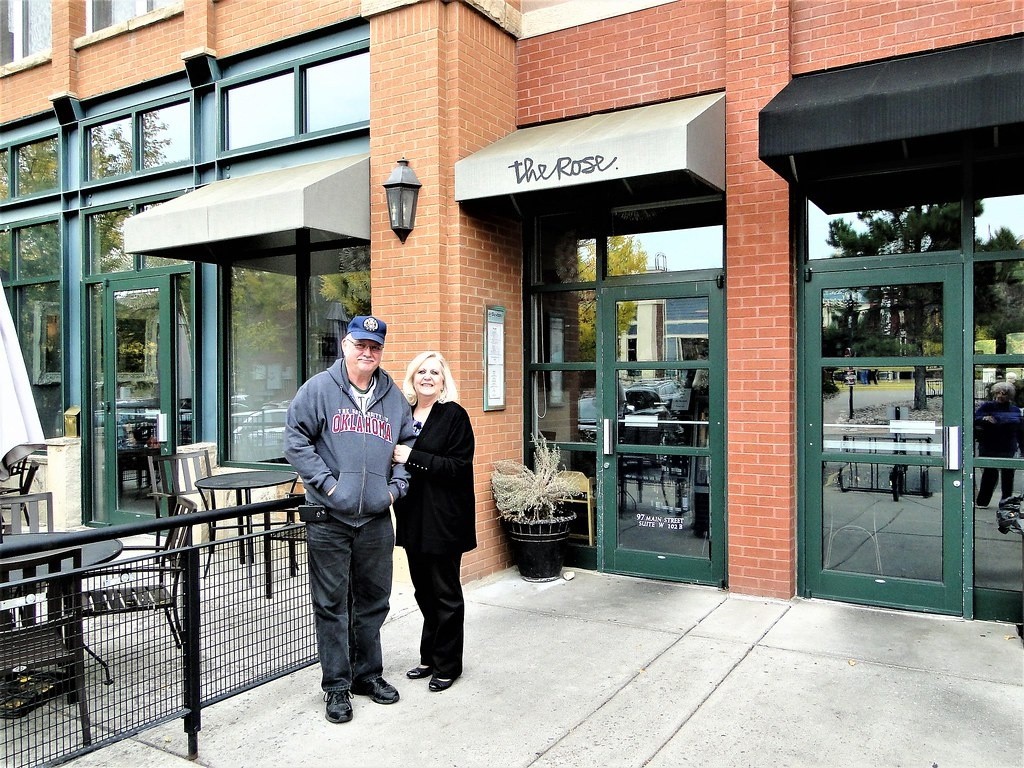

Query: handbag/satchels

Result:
[973,402,992,441]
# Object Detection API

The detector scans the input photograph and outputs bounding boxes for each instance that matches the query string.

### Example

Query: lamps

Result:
[382,157,422,244]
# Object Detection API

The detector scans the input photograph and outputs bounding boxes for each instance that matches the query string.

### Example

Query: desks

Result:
[195,470,298,588]
[0,533,123,704]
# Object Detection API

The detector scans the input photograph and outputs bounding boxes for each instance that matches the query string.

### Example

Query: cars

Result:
[230,393,292,444]
[100,395,193,448]
[578,379,682,443]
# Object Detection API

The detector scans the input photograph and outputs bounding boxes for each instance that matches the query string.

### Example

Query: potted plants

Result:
[491,429,583,577]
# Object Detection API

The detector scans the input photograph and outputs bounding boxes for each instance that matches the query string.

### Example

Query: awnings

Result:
[453,89,727,222]
[756,35,1024,182]
[124,152,372,267]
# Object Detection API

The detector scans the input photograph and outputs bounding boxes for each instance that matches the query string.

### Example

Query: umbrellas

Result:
[0,276,45,543]
[323,301,348,369]
[154,311,191,399]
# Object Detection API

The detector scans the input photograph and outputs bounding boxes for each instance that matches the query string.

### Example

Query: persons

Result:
[392,350,477,691]
[858,367,1024,509]
[674,357,710,538]
[285,316,417,721]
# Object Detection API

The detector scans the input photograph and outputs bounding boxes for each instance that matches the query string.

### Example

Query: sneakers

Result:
[351,675,400,705]
[324,690,355,723]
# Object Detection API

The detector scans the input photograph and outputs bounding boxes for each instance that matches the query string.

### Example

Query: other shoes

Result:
[975,503,989,509]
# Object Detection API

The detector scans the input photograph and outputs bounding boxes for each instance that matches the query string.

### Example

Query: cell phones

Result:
[299,505,328,521]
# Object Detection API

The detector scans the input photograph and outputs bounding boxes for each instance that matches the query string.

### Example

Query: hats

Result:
[347,316,386,345]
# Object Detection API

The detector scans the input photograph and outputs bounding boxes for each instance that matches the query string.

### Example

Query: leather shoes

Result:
[407,664,432,679]
[428,677,456,692]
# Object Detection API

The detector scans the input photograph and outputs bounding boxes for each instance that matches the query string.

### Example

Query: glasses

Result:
[347,339,384,352]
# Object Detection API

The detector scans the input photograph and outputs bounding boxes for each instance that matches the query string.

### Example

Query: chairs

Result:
[69,497,199,685]
[0,546,94,746]
[264,492,308,598]
[0,459,54,533]
[148,450,216,569]
